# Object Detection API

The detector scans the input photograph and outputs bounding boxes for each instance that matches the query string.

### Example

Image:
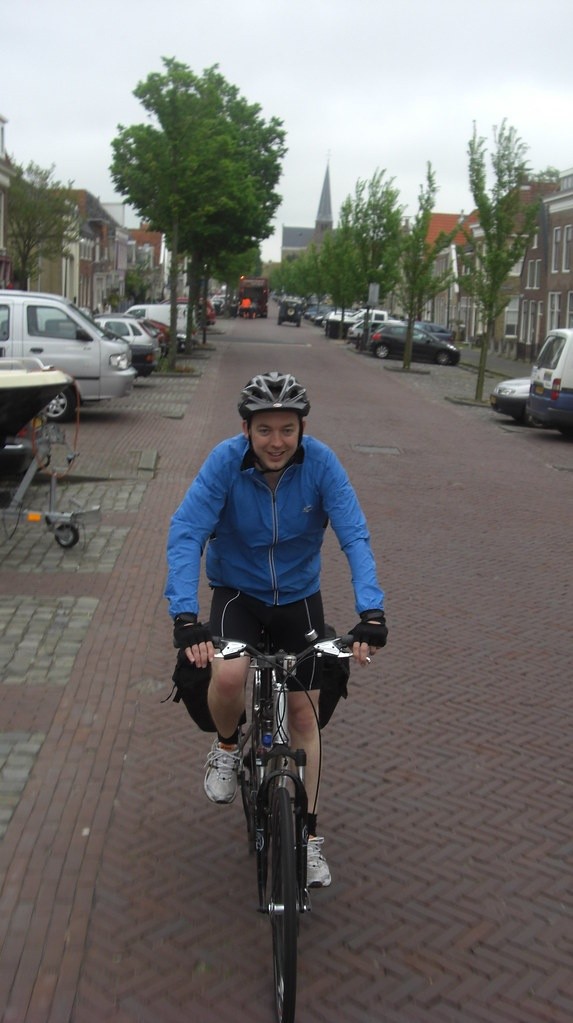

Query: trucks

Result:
[239,277,269,318]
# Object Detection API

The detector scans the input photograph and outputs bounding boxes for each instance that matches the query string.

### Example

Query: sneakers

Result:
[306,835,332,888]
[201,737,241,804]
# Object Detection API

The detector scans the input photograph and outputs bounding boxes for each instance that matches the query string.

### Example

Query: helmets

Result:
[238,371,311,420]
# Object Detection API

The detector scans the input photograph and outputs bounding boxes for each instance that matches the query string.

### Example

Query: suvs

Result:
[277,299,302,327]
[90,316,157,381]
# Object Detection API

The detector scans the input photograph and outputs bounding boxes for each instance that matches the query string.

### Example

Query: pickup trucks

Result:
[349,309,403,329]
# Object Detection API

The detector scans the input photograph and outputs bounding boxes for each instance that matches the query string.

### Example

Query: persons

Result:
[163,371,386,889]
[240,298,256,318]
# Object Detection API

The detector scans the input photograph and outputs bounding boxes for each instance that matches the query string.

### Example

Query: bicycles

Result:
[197,635,370,1023]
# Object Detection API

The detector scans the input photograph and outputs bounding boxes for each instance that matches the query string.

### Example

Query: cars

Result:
[323,308,353,326]
[369,325,461,366]
[349,320,417,341]
[490,378,557,429]
[209,294,237,314]
[302,304,330,324]
[134,318,169,357]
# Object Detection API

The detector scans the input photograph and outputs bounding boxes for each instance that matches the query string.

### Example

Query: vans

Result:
[123,304,195,346]
[158,298,217,328]
[526,328,573,441]
[0,288,138,421]
